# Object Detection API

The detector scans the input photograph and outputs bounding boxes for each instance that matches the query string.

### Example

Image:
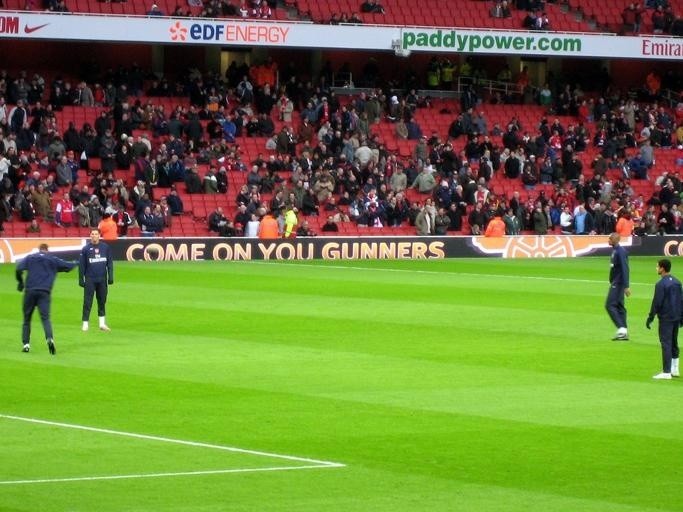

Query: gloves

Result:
[646,320,653,329]
[79,280,85,287]
[18,284,24,291]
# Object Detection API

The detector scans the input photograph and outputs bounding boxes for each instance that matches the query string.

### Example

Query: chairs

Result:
[1,0,682,237]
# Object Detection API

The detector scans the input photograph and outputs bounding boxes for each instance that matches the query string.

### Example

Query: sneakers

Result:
[101,325,110,331]
[47,336,55,355]
[612,332,629,340]
[82,321,88,331]
[653,372,672,379]
[671,369,679,376]
[23,347,29,352]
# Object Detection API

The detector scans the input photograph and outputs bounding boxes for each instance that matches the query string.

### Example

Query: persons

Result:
[15,243,76,355]
[79,227,114,331]
[2,57,682,241]
[2,1,387,27]
[645,260,683,380]
[605,232,631,341]
[490,0,683,41]
